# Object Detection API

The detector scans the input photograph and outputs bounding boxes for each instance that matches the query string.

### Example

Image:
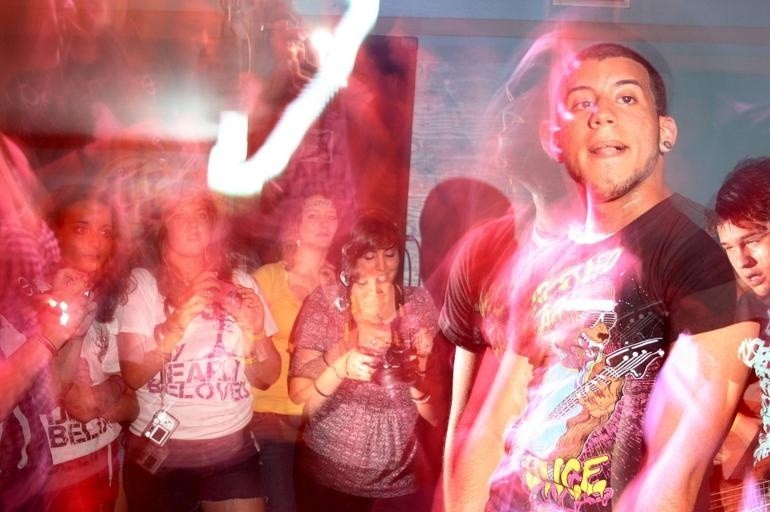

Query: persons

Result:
[1,21,770,511]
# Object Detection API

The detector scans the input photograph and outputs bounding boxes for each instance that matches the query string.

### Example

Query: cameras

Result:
[142,410,179,448]
[388,342,420,382]
